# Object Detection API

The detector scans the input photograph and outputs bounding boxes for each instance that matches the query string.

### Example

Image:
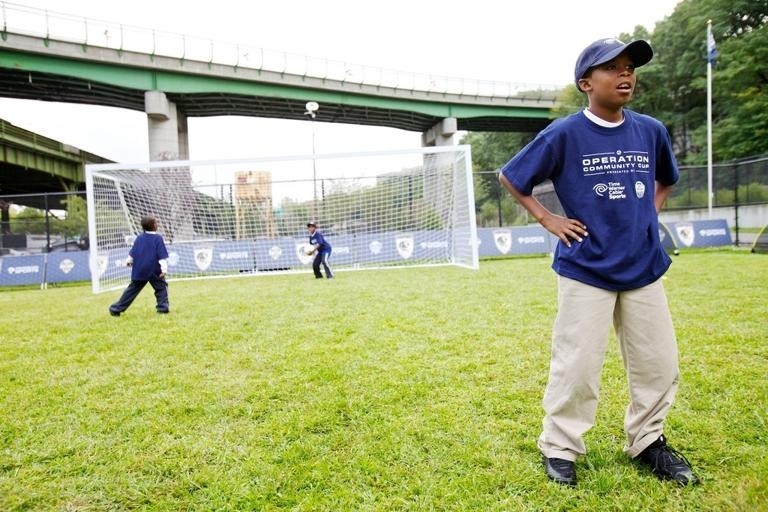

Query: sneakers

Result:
[637,431,704,491]
[542,453,579,490]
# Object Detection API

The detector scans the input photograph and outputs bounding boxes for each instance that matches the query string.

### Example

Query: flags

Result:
[707,30,720,65]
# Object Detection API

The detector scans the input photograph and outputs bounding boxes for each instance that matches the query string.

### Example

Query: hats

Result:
[572,34,656,86]
[306,221,317,227]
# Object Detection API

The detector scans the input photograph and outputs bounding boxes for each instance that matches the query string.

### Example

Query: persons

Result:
[497,36,701,489]
[109,216,170,317]
[306,222,334,281]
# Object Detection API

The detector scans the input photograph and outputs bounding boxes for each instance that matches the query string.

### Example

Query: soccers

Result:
[304,245,314,256]
